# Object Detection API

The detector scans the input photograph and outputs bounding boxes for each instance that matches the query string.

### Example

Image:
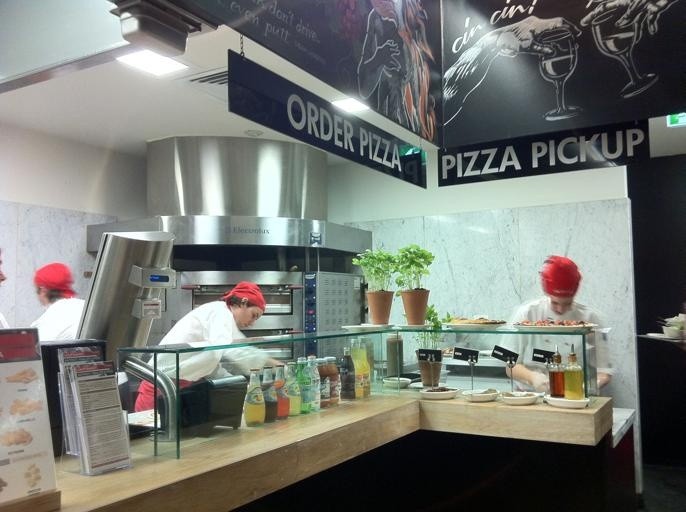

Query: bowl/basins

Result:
[661,326,685,340]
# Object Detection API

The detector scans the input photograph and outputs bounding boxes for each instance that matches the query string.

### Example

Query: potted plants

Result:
[395,241,434,326]
[404,305,453,386]
[350,244,396,327]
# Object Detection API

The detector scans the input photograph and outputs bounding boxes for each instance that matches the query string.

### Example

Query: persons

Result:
[501,255,615,394]
[582,2,676,45]
[132,281,286,413]
[442,14,582,127]
[25,262,87,342]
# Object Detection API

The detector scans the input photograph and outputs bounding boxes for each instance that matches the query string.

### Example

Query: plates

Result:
[513,324,597,335]
[336,324,395,333]
[498,392,538,405]
[383,377,411,389]
[396,323,430,330]
[544,396,590,409]
[459,388,497,402]
[420,387,457,400]
[440,322,504,331]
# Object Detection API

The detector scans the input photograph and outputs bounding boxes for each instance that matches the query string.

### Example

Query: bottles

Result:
[242,356,338,427]
[339,339,370,399]
[385,334,402,378]
[563,353,583,399]
[547,354,566,399]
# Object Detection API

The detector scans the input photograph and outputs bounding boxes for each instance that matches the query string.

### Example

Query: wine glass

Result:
[588,9,659,101]
[532,29,580,121]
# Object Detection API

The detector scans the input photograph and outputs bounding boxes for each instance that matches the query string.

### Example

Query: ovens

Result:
[178,271,306,365]
